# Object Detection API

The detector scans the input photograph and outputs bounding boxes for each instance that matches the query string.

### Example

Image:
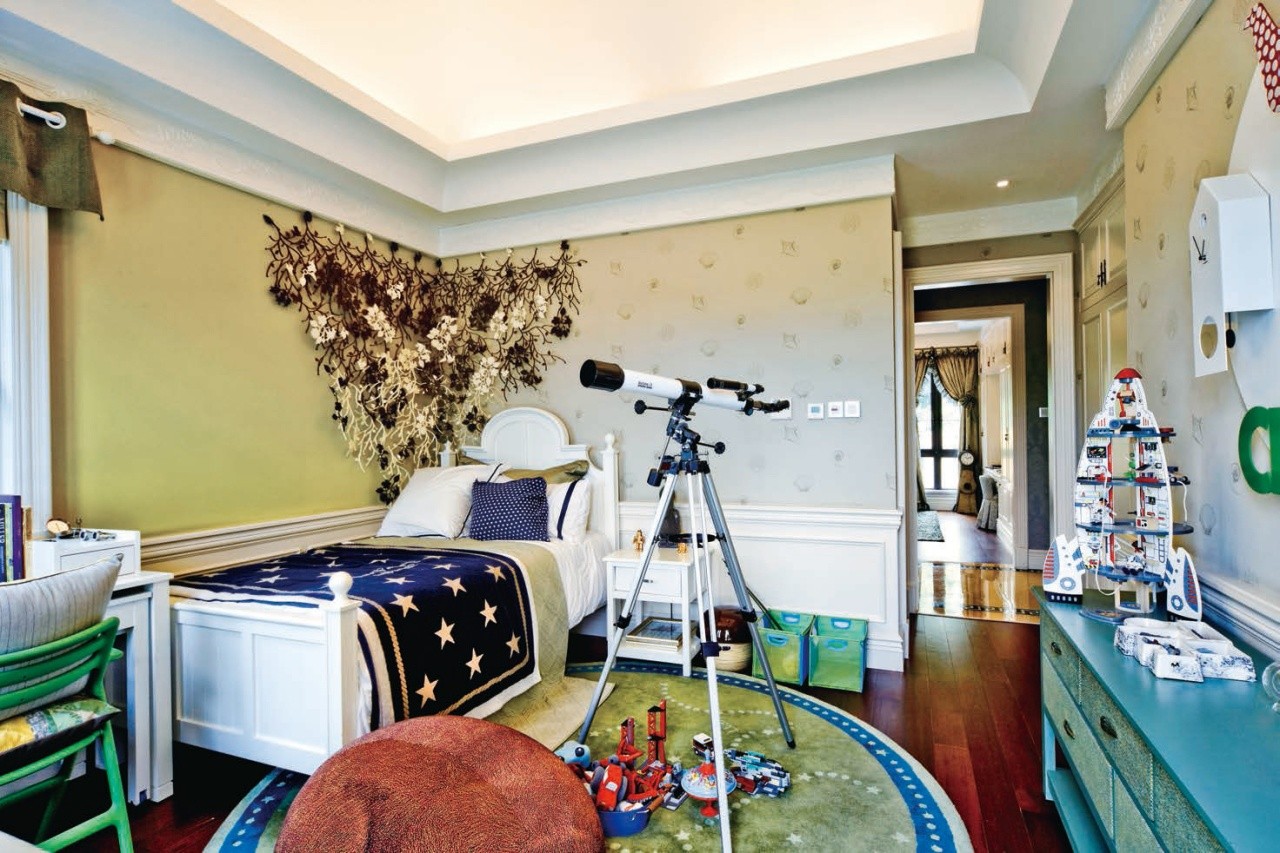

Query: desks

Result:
[1030,583,1280,853]
[100,570,176,804]
[985,466,1002,493]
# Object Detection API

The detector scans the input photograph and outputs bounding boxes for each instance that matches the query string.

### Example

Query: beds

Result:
[170,405,621,776]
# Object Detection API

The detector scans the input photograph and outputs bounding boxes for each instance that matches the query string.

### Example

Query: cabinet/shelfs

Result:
[1072,164,1127,312]
[1075,284,1128,470]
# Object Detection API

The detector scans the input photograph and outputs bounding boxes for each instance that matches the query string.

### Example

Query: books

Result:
[0,494,28,582]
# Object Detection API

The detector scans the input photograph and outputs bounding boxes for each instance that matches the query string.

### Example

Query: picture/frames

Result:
[625,616,698,652]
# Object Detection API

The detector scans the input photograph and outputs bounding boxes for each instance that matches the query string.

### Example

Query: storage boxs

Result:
[808,615,869,693]
[28,527,141,578]
[751,608,815,686]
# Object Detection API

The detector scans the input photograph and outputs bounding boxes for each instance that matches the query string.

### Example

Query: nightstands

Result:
[602,545,716,677]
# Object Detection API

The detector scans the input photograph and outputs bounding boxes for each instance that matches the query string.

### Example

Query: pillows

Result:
[0,551,124,722]
[470,476,550,543]
[375,463,504,539]
[461,460,592,544]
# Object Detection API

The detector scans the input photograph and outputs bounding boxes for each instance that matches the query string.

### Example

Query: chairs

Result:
[976,475,999,531]
[0,617,134,853]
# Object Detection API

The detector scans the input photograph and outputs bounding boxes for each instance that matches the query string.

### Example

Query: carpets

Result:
[200,661,976,853]
[916,511,945,541]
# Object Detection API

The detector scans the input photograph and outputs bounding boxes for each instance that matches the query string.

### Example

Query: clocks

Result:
[959,451,974,466]
[1189,173,1276,378]
